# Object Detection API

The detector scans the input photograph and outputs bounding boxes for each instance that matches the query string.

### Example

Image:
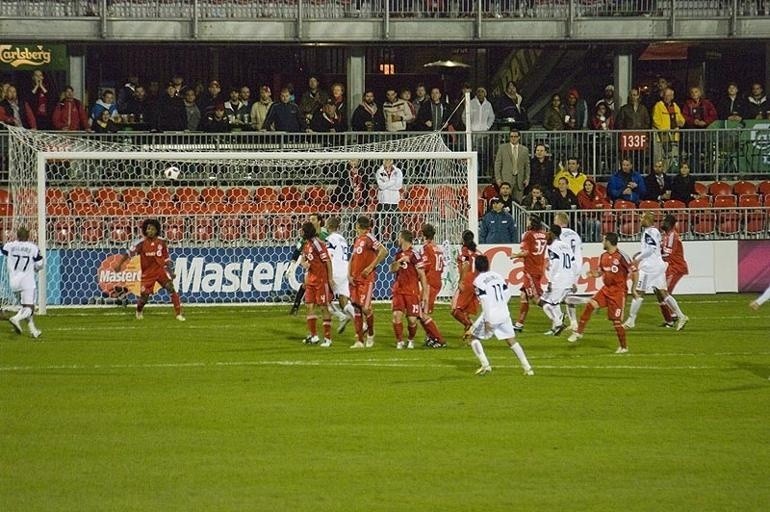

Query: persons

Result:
[331,159,403,243]
[114,219,186,321]
[479,130,697,245]
[286,214,535,376]
[337,0,540,18]
[1,75,769,187]
[1,226,44,338]
[510,213,689,354]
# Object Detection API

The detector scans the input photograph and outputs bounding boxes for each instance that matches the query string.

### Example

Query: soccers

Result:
[167,167,180,181]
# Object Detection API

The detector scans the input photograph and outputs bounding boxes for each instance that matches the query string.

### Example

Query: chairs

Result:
[0,176,770,253]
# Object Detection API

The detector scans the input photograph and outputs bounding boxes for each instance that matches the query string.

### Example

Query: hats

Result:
[208,79,220,89]
[164,81,175,89]
[259,85,271,93]
[323,96,335,106]
[726,81,737,87]
[506,81,516,87]
[214,103,224,113]
[491,195,502,204]
[605,84,615,92]
[476,86,488,96]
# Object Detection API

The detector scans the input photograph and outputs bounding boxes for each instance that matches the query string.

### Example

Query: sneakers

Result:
[301,334,319,344]
[471,366,492,377]
[622,323,636,329]
[350,341,364,349]
[176,315,185,321]
[520,370,535,378]
[366,331,376,348]
[511,317,584,344]
[32,330,42,339]
[135,310,143,320]
[354,322,369,338]
[663,313,690,332]
[9,317,22,334]
[425,338,449,349]
[336,317,351,334]
[394,339,416,350]
[615,346,631,354]
[320,336,333,348]
